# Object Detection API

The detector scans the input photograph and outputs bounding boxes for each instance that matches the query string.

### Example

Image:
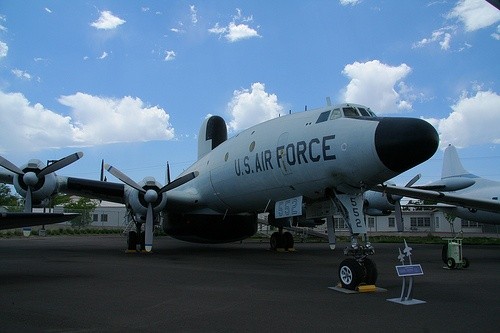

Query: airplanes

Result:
[2,96,500,293]
